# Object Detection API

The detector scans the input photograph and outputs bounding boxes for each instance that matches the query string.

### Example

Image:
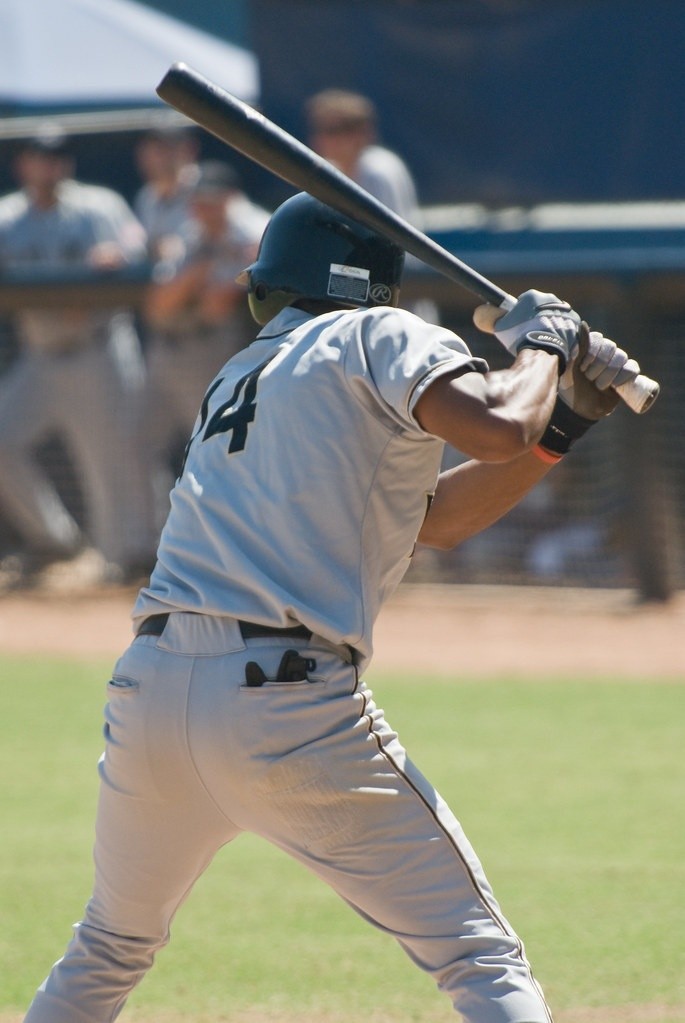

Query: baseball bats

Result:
[156,61,663,418]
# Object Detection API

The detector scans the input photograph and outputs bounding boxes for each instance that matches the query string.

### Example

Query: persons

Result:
[309,86,426,315]
[0,124,281,594]
[22,187,642,1023]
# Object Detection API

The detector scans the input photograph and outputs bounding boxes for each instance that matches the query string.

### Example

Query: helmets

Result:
[242,191,405,327]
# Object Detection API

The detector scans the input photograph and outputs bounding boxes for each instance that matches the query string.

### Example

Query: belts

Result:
[134,613,312,640]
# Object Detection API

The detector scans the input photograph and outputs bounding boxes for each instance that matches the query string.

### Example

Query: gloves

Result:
[557,321,641,422]
[473,290,581,378]
